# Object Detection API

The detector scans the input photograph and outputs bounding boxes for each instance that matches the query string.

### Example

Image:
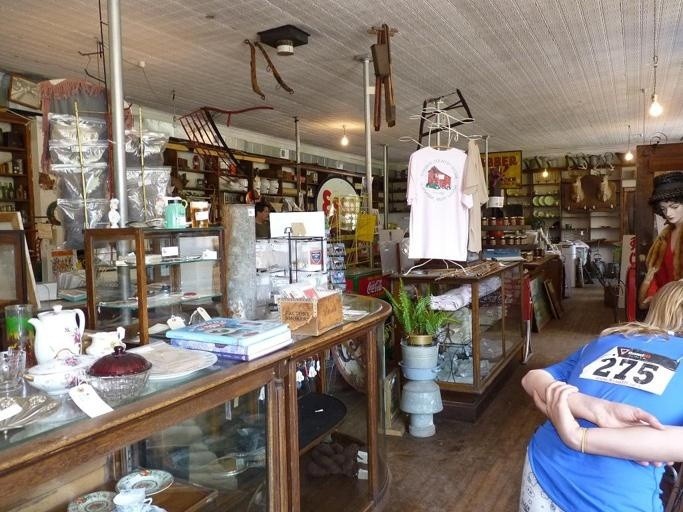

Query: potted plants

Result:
[380,278,463,347]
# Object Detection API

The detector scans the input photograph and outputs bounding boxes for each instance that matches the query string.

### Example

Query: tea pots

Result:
[85,326,126,357]
[27,303,86,366]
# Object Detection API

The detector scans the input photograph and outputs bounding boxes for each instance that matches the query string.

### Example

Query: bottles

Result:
[193,155,201,169]
[0,182,28,223]
[482,215,545,262]
[165,199,187,229]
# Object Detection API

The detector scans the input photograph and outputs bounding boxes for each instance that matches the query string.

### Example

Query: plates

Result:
[162,433,266,479]
[135,346,218,380]
[0,395,62,443]
[115,468,174,497]
[111,503,168,512]
[531,195,555,219]
[67,491,116,512]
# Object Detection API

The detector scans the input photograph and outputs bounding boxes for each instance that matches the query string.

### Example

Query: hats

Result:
[647,172,683,218]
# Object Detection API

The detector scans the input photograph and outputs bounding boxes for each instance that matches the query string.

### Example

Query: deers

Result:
[564,153,590,203]
[590,152,618,201]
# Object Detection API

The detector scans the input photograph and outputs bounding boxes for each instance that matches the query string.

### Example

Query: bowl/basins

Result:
[85,347,152,402]
[22,348,100,396]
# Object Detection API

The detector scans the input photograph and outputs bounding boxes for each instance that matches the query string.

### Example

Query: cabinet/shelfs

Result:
[391,261,527,426]
[82,228,226,345]
[490,166,621,285]
[0,289,392,511]
[0,107,39,262]
[162,136,409,228]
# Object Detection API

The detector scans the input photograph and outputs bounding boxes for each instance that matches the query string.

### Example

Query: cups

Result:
[112,488,153,512]
[0,350,27,400]
[4,303,35,370]
[189,201,211,228]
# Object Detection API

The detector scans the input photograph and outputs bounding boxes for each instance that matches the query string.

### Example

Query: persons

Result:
[636,172,682,307]
[517,278,683,512]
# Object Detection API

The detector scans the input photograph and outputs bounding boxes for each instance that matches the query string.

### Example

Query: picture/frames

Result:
[0,210,40,307]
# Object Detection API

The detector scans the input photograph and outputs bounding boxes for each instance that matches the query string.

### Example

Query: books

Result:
[169,331,294,356]
[215,340,293,361]
[164,316,290,347]
